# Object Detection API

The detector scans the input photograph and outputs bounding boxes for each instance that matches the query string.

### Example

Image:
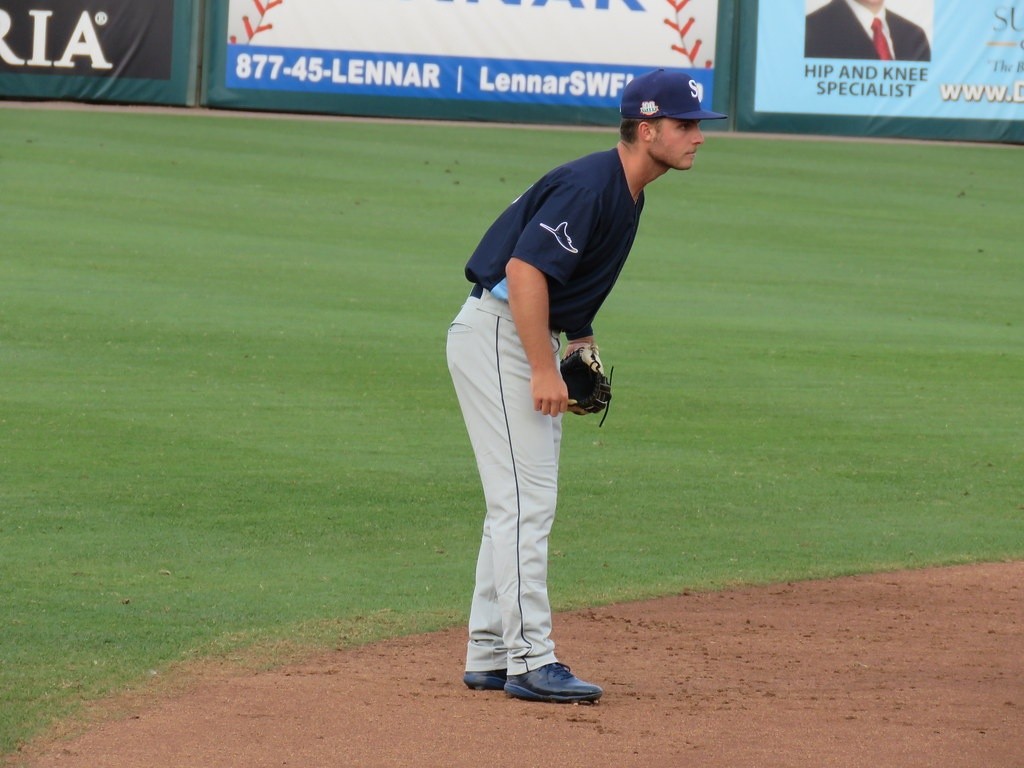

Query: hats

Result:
[620,67,729,120]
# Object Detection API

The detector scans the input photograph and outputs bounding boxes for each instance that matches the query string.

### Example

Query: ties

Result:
[870,18,893,59]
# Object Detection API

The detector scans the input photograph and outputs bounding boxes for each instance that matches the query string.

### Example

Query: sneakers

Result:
[460,666,505,692]
[506,662,604,700]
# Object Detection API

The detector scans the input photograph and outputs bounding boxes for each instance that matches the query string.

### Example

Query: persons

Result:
[446,70,728,705]
[806,0,929,59]
